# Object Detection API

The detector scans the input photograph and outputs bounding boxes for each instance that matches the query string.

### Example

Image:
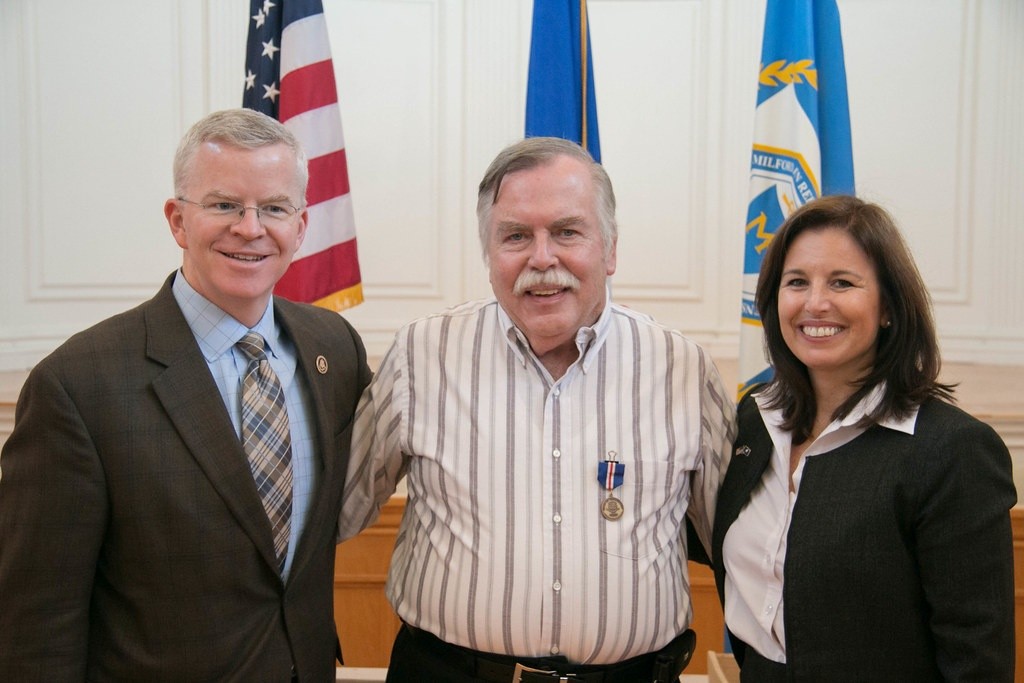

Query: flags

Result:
[730,0,858,416]
[523,0,603,168]
[241,0,366,314]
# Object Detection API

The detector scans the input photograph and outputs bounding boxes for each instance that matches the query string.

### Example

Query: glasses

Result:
[177,193,308,227]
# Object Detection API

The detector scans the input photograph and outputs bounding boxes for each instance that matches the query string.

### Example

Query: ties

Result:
[232,331,295,581]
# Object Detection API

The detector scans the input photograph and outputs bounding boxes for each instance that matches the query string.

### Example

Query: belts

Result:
[390,623,697,683]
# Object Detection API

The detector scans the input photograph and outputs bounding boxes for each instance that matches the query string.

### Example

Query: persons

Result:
[683,195,1019,683]
[334,135,740,683]
[1,107,378,683]
